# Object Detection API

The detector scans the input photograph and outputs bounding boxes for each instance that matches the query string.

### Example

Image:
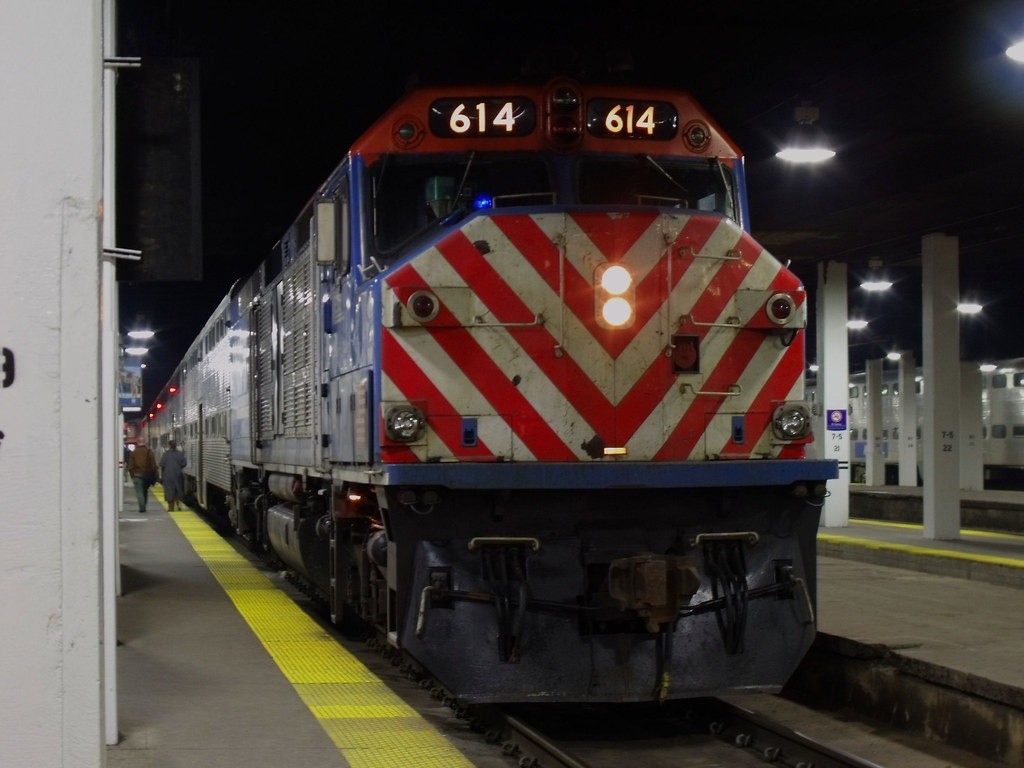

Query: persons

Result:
[125,436,156,512]
[159,440,187,512]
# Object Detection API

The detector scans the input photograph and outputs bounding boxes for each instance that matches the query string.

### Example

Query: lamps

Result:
[777,100,837,173]
[315,190,344,267]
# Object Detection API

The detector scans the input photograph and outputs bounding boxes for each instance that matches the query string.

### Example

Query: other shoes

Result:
[139,507,146,512]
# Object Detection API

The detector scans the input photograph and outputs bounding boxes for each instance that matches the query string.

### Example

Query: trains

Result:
[800,354,1023,491]
[133,84,844,728]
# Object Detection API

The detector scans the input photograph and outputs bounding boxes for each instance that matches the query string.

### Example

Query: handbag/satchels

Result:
[147,468,159,486]
